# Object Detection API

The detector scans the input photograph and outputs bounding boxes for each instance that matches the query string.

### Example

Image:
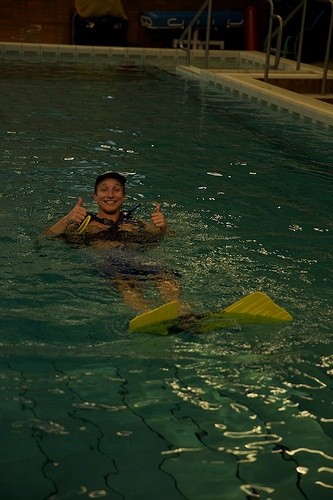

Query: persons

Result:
[49,172,169,234]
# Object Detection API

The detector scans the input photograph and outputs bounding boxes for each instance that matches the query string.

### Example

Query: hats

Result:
[94,170,126,182]
